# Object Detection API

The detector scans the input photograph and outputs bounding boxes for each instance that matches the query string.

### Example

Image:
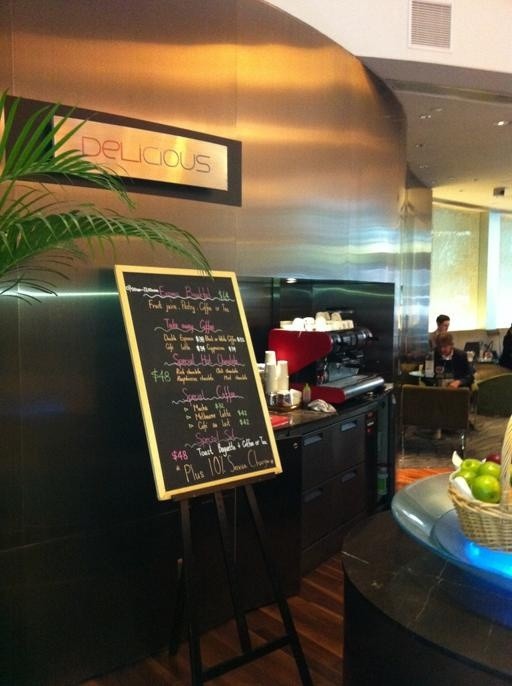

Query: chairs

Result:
[395,349,512,458]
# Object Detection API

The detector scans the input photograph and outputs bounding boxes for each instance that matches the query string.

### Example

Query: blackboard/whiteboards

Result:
[113,264,283,503]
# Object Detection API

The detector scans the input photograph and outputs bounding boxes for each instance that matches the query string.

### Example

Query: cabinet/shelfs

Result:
[249,383,396,600]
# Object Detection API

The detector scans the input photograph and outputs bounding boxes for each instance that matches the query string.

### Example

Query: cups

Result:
[435,365,445,373]
[279,311,355,332]
[262,351,302,407]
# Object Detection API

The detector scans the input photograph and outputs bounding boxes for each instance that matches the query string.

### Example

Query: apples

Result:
[456,452,512,503]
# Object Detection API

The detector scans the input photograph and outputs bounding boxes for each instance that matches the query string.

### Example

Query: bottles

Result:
[302,382,312,404]
[425,338,436,378]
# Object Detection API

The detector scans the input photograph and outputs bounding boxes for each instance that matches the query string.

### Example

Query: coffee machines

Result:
[266,323,385,404]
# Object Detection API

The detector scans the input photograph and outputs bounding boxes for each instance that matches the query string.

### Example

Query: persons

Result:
[429,315,450,351]
[432,332,475,389]
[499,322,512,369]
